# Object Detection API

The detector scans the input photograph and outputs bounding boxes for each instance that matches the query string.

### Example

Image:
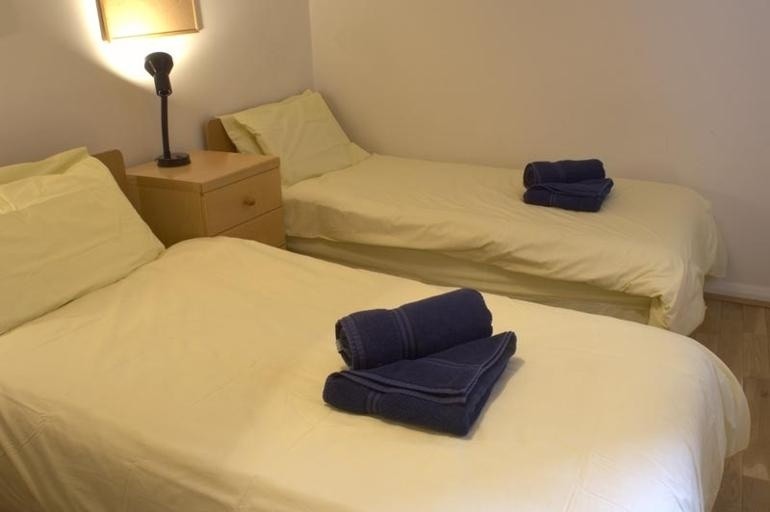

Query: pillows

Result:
[236,91,369,185]
[215,90,312,152]
[2,157,164,334]
[0,146,85,183]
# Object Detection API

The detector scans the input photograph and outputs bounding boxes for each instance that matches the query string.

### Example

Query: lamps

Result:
[144,52,190,167]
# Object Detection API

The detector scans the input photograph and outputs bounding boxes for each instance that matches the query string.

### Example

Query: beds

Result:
[1,148,750,511]
[205,87,725,336]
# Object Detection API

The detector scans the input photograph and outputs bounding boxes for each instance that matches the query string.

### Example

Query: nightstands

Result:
[124,149,288,248]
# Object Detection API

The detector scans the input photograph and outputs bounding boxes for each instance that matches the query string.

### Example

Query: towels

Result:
[523,159,606,187]
[323,332,516,435]
[335,287,492,369]
[522,177,613,212]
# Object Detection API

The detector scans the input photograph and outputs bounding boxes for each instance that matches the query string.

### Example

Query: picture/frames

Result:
[95,0,204,42]
